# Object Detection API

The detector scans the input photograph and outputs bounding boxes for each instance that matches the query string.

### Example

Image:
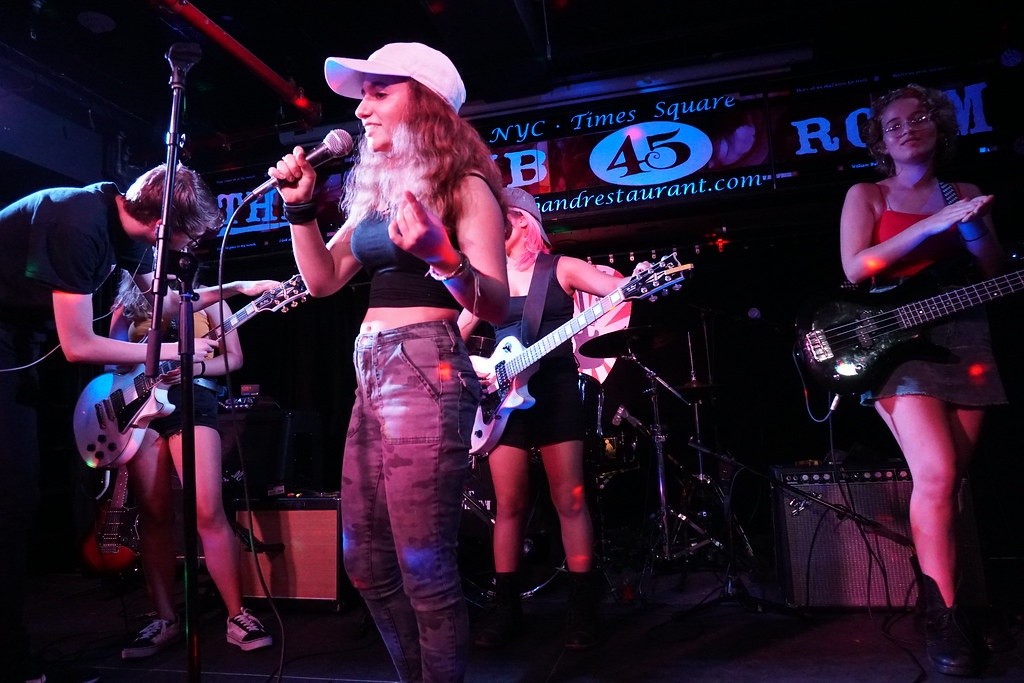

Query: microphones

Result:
[522,538,537,559]
[612,404,624,425]
[244,129,354,205]
[747,308,788,337]
[243,542,285,554]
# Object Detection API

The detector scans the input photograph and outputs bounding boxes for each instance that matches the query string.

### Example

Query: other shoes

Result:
[40,667,100,683]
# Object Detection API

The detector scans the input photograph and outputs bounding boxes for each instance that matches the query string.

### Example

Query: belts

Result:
[192,378,219,392]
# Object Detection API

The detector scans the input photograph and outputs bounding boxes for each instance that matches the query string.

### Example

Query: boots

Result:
[471,578,525,647]
[563,575,610,649]
[910,552,1017,675]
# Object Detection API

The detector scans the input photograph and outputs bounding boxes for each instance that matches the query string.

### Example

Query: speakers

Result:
[236,496,345,608]
[213,409,324,522]
[773,467,990,615]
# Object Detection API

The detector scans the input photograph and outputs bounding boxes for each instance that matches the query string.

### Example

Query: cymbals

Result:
[675,382,731,389]
[579,326,672,360]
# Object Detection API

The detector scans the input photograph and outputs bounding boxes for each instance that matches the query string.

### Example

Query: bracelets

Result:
[430,249,470,281]
[282,201,318,225]
[197,361,206,378]
[957,217,990,243]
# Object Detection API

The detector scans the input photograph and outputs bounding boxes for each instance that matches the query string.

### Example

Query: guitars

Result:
[465,249,696,455]
[789,270,1023,422]
[79,465,141,573]
[71,274,309,470]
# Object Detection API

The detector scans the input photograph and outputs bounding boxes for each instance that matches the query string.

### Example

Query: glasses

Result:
[176,225,201,250]
[884,114,931,136]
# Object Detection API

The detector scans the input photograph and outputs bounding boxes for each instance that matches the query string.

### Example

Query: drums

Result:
[527,370,604,464]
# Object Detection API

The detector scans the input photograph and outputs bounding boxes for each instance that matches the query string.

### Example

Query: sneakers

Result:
[227,606,272,650]
[121,618,180,658]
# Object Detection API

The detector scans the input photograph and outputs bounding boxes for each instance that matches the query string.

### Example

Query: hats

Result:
[502,186,542,224]
[324,41,466,116]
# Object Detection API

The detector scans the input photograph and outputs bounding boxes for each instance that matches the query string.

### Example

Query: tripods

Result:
[611,318,913,632]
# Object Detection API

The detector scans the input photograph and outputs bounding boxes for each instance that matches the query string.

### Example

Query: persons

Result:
[0,159,287,683]
[456,187,655,652]
[270,41,510,683]
[839,82,1005,675]
[102,241,272,657]
